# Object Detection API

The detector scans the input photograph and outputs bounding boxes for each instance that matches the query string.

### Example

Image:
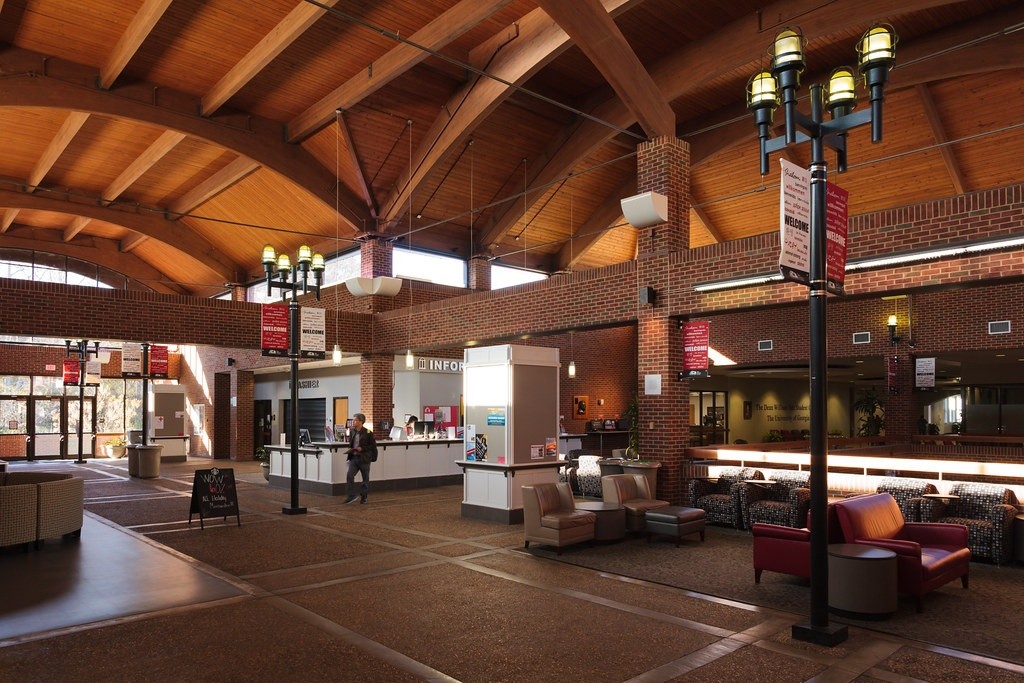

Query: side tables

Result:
[828,544,898,623]
[574,501,626,544]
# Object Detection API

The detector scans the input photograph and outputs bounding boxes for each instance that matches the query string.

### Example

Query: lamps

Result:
[405,118,416,371]
[333,106,342,367]
[886,314,903,347]
[567,331,576,379]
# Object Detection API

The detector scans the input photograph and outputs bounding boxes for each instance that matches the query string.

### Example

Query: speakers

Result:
[227,358,232,366]
[641,288,654,304]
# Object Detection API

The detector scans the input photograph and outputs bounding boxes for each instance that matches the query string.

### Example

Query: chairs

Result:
[769,429,810,442]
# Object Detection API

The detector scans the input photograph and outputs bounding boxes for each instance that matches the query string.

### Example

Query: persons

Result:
[344,414,377,504]
[917,415,927,443]
[409,416,418,427]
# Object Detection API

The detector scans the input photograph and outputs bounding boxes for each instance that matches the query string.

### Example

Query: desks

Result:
[585,430,632,457]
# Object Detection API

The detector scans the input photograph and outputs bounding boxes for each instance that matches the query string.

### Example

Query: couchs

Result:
[751,491,878,585]
[601,474,670,540]
[834,492,970,615]
[739,469,811,531]
[687,466,765,532]
[566,448,600,494]
[0,471,85,553]
[520,482,596,556]
[575,454,608,499]
[919,482,1022,568]
[845,476,939,522]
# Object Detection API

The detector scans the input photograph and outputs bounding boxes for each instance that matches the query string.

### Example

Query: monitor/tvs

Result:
[299,429,311,447]
[414,421,435,439]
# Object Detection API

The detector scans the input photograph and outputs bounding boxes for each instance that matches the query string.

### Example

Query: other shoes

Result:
[345,496,357,504]
[361,498,367,504]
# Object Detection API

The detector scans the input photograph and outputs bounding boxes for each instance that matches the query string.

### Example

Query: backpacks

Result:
[367,429,377,462]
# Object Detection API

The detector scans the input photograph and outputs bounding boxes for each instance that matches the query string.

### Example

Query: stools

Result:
[644,505,706,548]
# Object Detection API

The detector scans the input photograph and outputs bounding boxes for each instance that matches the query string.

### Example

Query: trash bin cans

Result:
[625,457,661,500]
[596,457,623,475]
[136,444,164,478]
[124,444,139,476]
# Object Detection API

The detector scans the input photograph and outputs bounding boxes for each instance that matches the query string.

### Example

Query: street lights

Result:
[747,23,897,646]
[263,243,326,515]
[64,338,100,464]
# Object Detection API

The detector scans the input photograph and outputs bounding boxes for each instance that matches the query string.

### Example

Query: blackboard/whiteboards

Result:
[190,468,239,519]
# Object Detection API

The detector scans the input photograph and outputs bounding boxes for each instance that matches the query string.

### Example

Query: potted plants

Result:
[256,447,270,481]
[103,437,128,460]
